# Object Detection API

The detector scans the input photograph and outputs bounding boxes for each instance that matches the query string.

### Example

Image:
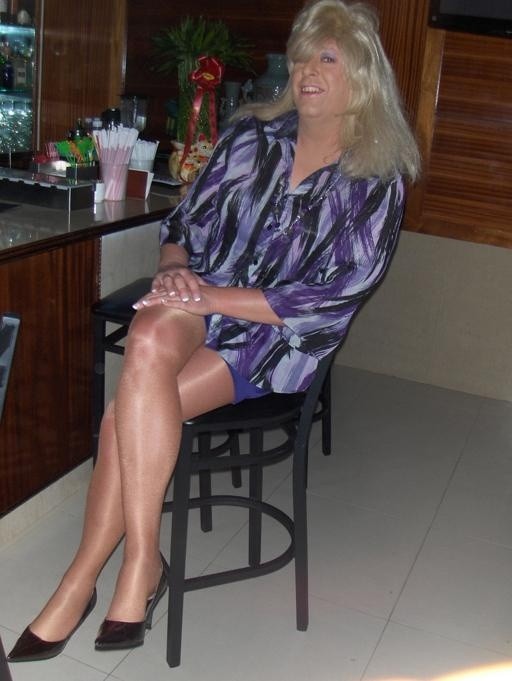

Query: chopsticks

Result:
[91,124,159,201]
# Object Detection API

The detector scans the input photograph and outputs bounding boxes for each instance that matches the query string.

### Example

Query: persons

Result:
[6,0,422,663]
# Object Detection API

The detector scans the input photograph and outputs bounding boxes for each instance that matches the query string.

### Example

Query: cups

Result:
[103,198,127,222]
[92,180,105,204]
[98,161,131,202]
[131,160,154,173]
[94,202,103,223]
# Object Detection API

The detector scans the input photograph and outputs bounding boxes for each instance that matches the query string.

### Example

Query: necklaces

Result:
[276,175,343,244]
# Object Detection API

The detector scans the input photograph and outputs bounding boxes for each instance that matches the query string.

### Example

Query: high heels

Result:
[95,550,170,651]
[6,588,97,661]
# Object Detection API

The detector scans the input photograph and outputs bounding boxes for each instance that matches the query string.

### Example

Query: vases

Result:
[241,53,289,104]
[219,81,242,121]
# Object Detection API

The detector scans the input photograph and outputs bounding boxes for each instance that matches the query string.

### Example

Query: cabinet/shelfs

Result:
[0,1,44,97]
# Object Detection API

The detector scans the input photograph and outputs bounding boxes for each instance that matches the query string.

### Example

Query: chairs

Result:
[146,349,335,667]
[0,313,21,424]
[88,275,333,488]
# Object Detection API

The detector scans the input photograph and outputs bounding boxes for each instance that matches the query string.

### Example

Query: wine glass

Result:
[0,97,35,152]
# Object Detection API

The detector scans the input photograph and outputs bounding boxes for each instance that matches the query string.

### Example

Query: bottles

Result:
[121,38,293,144]
[0,36,35,93]
[64,116,104,144]
